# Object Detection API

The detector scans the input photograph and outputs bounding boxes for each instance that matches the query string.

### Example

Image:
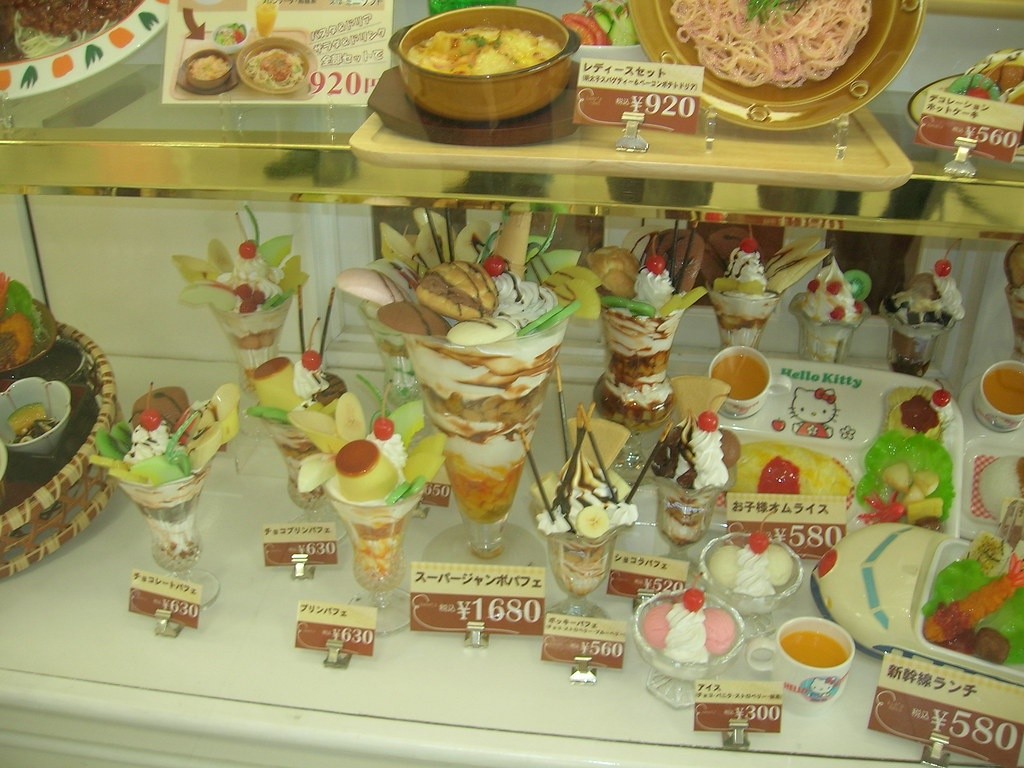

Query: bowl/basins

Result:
[0,375,71,453]
[570,43,649,64]
[180,49,235,89]
[236,38,318,95]
[0,299,57,370]
[212,22,251,54]
[388,5,582,121]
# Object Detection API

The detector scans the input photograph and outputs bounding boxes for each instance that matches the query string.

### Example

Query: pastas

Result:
[14,10,123,58]
[669,0,873,88]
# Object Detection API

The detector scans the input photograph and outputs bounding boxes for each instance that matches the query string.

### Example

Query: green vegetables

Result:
[743,0,806,24]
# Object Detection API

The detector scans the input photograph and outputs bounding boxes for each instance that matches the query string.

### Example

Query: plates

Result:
[0,0,169,99]
[916,539,1023,680]
[626,0,924,132]
[905,74,1024,162]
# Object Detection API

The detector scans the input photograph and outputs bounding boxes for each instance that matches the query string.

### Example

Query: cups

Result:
[787,290,865,365]
[974,355,1023,430]
[257,0,277,40]
[708,348,771,423]
[748,616,855,714]
[881,292,952,378]
[710,283,780,354]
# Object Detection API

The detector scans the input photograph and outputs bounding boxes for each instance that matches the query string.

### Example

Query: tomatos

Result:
[561,14,606,46]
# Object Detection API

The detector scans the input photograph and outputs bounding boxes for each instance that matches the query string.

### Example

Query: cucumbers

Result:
[592,7,638,45]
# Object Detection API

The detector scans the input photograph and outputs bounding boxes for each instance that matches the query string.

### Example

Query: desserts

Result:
[81,203,965,676]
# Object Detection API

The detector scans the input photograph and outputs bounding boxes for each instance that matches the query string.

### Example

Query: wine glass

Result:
[115,458,220,608]
[633,595,743,706]
[206,274,778,636]
[700,525,805,639]
